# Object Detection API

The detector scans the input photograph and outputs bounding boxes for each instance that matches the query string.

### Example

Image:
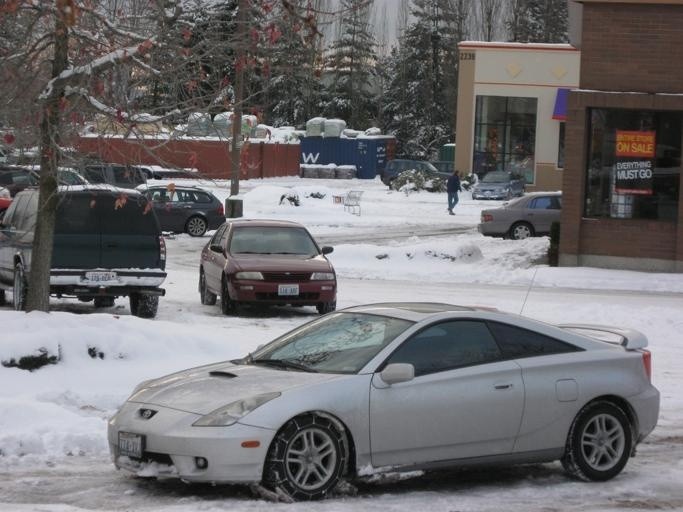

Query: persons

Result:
[446,169,462,215]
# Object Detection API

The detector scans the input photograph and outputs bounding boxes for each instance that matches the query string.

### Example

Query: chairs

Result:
[342,189,365,217]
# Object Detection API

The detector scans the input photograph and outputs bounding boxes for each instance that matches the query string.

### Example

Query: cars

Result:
[588,142,680,195]
[197,217,338,315]
[106,265,659,504]
[477,190,562,240]
[432,160,454,173]
[472,170,526,201]
[0,163,225,318]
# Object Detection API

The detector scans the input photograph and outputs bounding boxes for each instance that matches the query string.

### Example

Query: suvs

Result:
[379,158,453,191]
